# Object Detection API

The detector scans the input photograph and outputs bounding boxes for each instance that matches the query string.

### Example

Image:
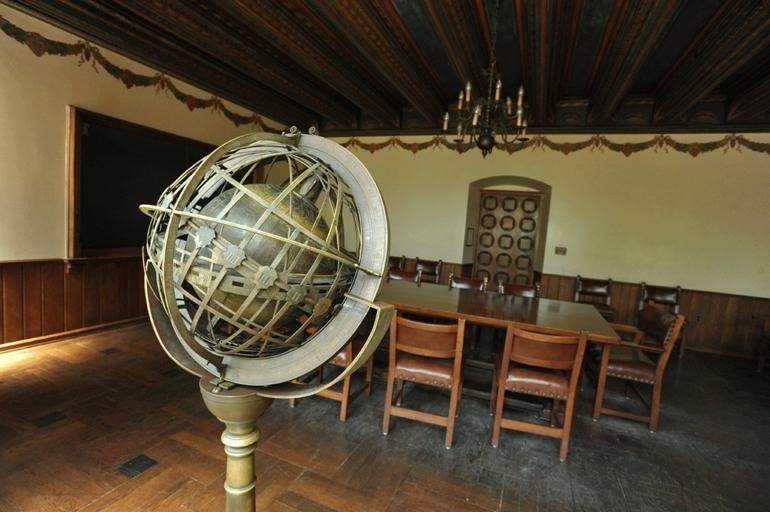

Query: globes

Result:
[139,126,396,512]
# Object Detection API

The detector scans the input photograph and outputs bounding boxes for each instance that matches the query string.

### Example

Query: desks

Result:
[377,279,620,433]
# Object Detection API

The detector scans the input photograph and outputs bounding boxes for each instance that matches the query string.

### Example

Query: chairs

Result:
[381,309,467,450]
[594,301,685,432]
[287,311,375,422]
[489,320,590,462]
[385,252,684,361]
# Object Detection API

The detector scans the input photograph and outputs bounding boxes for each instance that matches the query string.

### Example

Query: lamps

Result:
[440,0,529,157]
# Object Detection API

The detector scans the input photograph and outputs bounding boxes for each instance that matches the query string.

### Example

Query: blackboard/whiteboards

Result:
[64,104,257,261]
